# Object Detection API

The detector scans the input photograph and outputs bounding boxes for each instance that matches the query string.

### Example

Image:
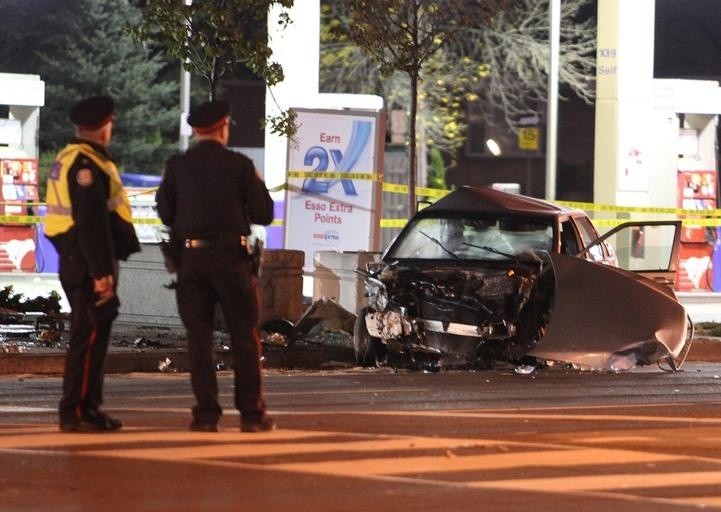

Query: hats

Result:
[187,101,237,134]
[70,96,116,130]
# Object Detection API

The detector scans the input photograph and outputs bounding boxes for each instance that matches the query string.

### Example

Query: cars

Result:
[355,185,692,373]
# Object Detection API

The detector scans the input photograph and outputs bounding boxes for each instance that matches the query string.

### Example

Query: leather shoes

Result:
[59,411,122,432]
[191,419,217,431]
[242,414,276,432]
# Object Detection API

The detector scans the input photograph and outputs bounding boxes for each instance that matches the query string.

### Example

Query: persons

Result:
[683,173,717,240]
[155,100,276,432]
[43,96,141,431]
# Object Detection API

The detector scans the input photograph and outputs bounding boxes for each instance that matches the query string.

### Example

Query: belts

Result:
[184,236,247,249]
